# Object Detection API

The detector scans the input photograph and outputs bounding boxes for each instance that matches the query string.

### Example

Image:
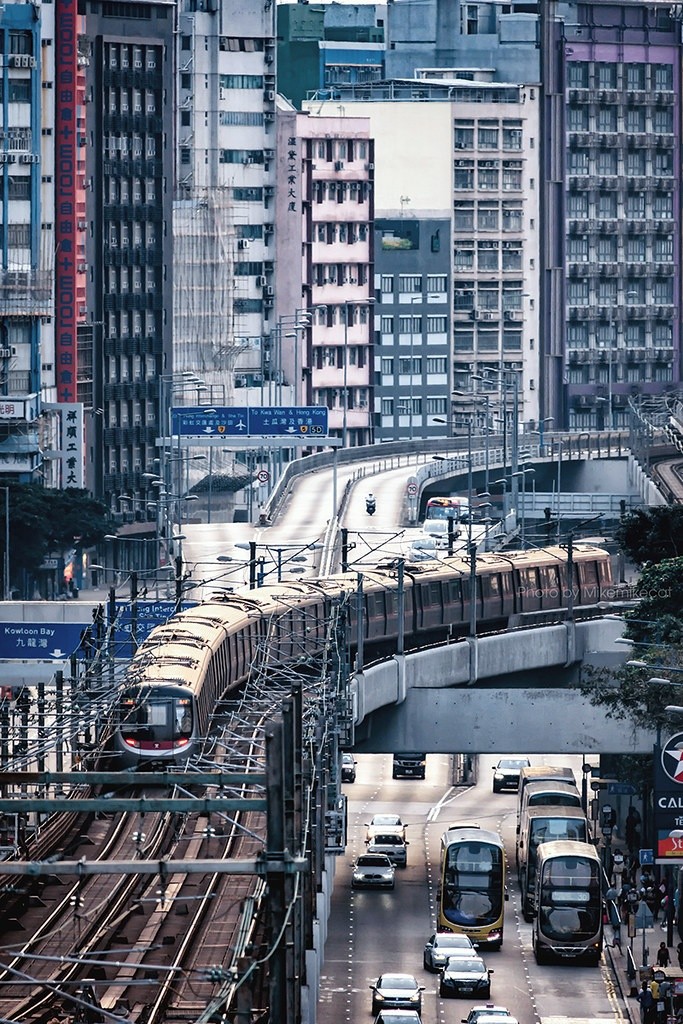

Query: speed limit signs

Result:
[257,471,269,482]
[408,484,417,494]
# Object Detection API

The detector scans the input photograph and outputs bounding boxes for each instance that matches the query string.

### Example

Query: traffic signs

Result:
[168,407,329,439]
[0,622,98,662]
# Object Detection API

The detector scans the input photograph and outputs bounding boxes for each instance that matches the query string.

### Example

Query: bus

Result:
[434,823,510,951]
[516,766,605,968]
[425,497,461,540]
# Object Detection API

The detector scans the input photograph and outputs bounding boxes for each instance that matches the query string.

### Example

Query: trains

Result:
[116,537,625,777]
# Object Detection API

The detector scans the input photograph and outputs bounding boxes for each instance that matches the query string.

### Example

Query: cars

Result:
[461,1003,520,1024]
[369,973,426,1024]
[423,930,494,998]
[340,753,358,783]
[363,814,409,869]
[492,758,531,793]
[409,538,438,562]
[349,853,397,890]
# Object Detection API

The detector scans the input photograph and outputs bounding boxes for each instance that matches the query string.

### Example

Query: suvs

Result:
[420,519,452,544]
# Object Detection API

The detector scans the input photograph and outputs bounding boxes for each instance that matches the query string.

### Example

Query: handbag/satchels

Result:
[661,898,666,907]
[657,1002,664,1011]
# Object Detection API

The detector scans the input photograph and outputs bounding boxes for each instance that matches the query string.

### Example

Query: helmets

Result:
[369,493,373,497]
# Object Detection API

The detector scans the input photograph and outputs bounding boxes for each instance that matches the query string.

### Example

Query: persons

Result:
[614,848,640,885]
[636,978,683,1024]
[367,493,375,500]
[618,871,679,927]
[605,882,618,922]
[61,577,74,599]
[625,806,641,853]
[657,942,671,967]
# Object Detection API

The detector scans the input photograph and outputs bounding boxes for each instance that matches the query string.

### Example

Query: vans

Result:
[392,752,426,779]
[451,496,474,524]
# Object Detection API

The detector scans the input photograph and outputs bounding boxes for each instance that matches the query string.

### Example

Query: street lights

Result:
[500,294,530,393]
[608,292,638,430]
[409,295,441,439]
[215,542,324,588]
[89,372,217,660]
[262,305,326,408]
[223,448,274,522]
[342,297,377,448]
[431,366,564,555]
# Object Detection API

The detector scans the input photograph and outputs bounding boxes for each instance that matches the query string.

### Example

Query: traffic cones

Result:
[627,971,639,998]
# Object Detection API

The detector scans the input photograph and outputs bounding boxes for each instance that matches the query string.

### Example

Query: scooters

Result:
[363,496,377,516]
